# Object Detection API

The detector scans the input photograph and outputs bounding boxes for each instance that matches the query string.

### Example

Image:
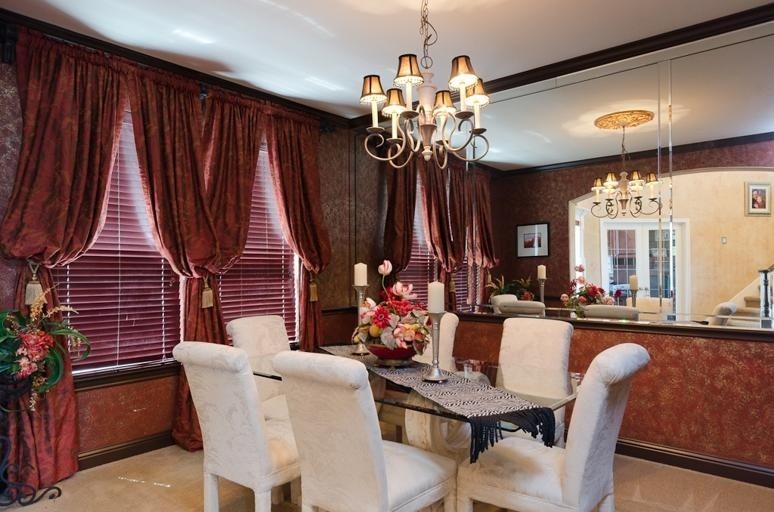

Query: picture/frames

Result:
[744,179,772,217]
[513,221,551,258]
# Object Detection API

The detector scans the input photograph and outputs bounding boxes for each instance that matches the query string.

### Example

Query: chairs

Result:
[271,351,456,512]
[497,299,546,316]
[440,317,574,453]
[489,294,518,314]
[583,304,639,321]
[456,342,650,512]
[702,300,737,326]
[171,340,301,511]
[384,312,459,422]
[626,296,673,321]
[224,316,295,420]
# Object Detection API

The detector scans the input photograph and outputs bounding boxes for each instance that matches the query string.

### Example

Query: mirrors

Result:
[353,1,773,332]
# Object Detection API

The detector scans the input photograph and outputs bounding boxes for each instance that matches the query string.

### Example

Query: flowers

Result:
[0,279,90,415]
[561,264,622,317]
[481,272,535,301]
[353,258,433,357]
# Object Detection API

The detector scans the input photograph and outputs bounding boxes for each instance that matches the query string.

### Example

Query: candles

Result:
[536,265,547,279]
[427,281,445,313]
[629,274,638,290]
[354,262,369,285]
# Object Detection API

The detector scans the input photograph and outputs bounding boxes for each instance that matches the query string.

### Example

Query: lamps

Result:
[355,0,492,172]
[590,109,664,220]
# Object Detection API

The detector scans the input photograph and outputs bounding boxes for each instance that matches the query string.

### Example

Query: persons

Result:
[751,189,766,209]
